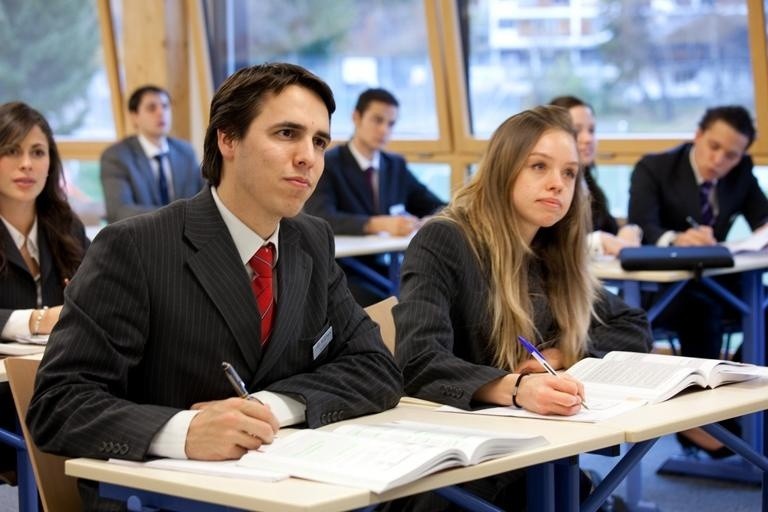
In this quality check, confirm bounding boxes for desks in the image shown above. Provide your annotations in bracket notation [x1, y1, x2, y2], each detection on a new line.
[400, 353, 766, 512]
[590, 247, 767, 482]
[64, 407, 628, 511]
[332, 231, 414, 298]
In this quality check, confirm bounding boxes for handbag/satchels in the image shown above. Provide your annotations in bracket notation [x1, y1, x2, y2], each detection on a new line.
[619, 245, 735, 270]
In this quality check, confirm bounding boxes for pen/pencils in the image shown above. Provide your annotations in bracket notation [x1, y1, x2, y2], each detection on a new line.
[222, 362, 253, 400]
[65, 279, 69, 283]
[518, 336, 590, 409]
[688, 216, 699, 228]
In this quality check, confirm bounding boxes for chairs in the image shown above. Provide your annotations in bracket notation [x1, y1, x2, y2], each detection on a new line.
[363, 295, 398, 356]
[2, 357, 83, 512]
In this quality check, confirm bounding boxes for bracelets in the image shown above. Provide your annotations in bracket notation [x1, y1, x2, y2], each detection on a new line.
[513, 373, 530, 409]
[34, 305, 48, 336]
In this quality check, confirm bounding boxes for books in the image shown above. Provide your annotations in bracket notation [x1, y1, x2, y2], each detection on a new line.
[560, 349, 762, 404]
[254, 410, 550, 498]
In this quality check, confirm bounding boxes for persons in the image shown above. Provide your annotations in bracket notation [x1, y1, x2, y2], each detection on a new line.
[548, 94, 747, 462]
[391, 106, 652, 419]
[300, 84, 454, 304]
[101, 79, 208, 231]
[24, 62, 407, 461]
[626, 103, 768, 366]
[0, 97, 98, 510]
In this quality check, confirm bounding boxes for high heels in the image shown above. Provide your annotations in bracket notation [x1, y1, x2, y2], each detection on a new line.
[676, 433, 734, 461]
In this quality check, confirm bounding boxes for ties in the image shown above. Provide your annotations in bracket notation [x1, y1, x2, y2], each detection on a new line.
[701, 181, 715, 225]
[154, 154, 170, 207]
[368, 168, 379, 213]
[248, 246, 274, 349]
